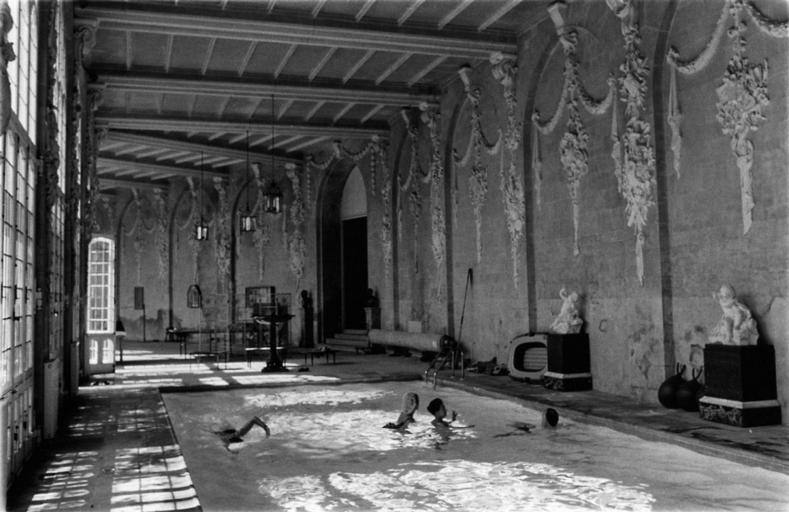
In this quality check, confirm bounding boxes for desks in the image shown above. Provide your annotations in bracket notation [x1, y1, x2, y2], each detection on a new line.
[171, 324, 271, 361]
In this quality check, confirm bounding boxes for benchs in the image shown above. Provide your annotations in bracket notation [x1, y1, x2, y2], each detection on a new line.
[298, 347, 341, 364]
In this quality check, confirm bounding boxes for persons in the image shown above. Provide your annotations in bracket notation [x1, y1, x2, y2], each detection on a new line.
[548, 286, 582, 336]
[425, 397, 476, 441]
[382, 389, 420, 435]
[539, 406, 568, 431]
[213, 415, 271, 454]
[716, 284, 754, 345]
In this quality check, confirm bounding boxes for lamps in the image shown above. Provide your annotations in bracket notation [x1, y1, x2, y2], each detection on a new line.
[194, 92, 283, 242]
